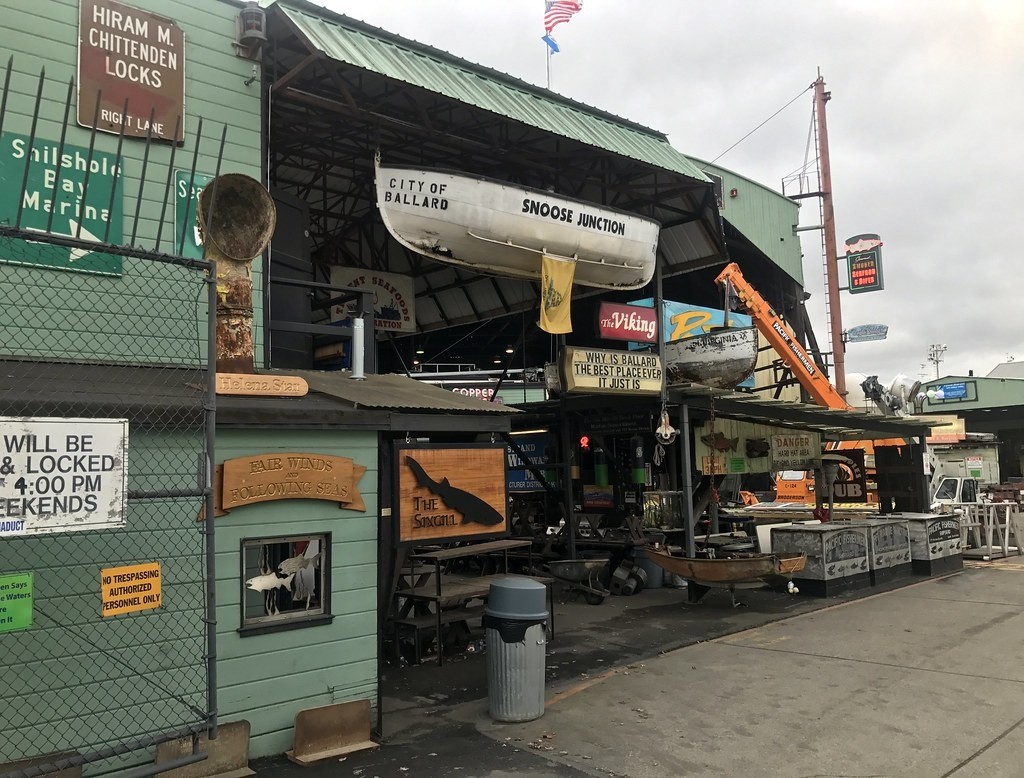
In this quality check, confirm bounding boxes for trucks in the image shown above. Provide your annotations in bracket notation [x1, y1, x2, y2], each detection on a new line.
[930, 476, 1018, 523]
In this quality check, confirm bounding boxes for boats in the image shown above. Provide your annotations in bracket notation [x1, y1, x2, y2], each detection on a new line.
[641, 545, 807, 581]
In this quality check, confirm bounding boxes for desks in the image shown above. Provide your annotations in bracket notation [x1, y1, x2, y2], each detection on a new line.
[868, 512, 963, 576]
[770, 527, 869, 598]
[831, 520, 913, 587]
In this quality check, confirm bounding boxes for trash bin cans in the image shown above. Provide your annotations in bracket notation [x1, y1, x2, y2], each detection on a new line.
[484, 577, 549, 723]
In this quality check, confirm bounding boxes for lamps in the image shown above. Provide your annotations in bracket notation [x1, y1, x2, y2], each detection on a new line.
[234, 3, 269, 59]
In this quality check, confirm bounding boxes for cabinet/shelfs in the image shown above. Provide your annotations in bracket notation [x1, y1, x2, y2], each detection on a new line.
[394, 540, 555, 666]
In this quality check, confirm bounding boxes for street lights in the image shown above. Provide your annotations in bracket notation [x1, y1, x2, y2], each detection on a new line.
[928, 344, 947, 379]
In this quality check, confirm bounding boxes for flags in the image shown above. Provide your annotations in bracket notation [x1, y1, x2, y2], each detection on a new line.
[330, 265, 416, 331]
[544, 0, 583, 32]
[536, 255, 576, 335]
